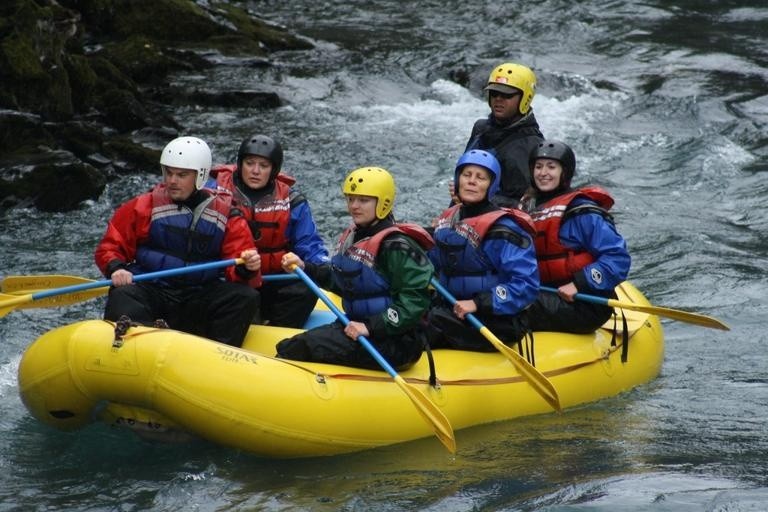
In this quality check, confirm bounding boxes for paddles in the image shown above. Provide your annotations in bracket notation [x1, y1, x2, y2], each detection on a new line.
[0, 255, 246, 320]
[292, 264, 457, 455]
[542, 288, 731, 332]
[432, 277, 563, 417]
[2, 273, 296, 308]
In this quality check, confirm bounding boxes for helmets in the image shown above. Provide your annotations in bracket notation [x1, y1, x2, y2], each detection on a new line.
[237, 132, 284, 188]
[342, 165, 397, 220]
[486, 62, 538, 115]
[454, 148, 500, 202]
[528, 140, 575, 183]
[159, 136, 213, 191]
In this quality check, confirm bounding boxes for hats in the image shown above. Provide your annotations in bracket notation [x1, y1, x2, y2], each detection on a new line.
[486, 82, 519, 95]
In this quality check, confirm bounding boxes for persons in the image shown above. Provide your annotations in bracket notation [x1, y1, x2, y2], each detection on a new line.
[203, 133, 333, 328]
[525, 141, 632, 364]
[274, 166, 437, 387]
[95, 135, 262, 350]
[448, 63, 547, 211]
[425, 150, 539, 371]
